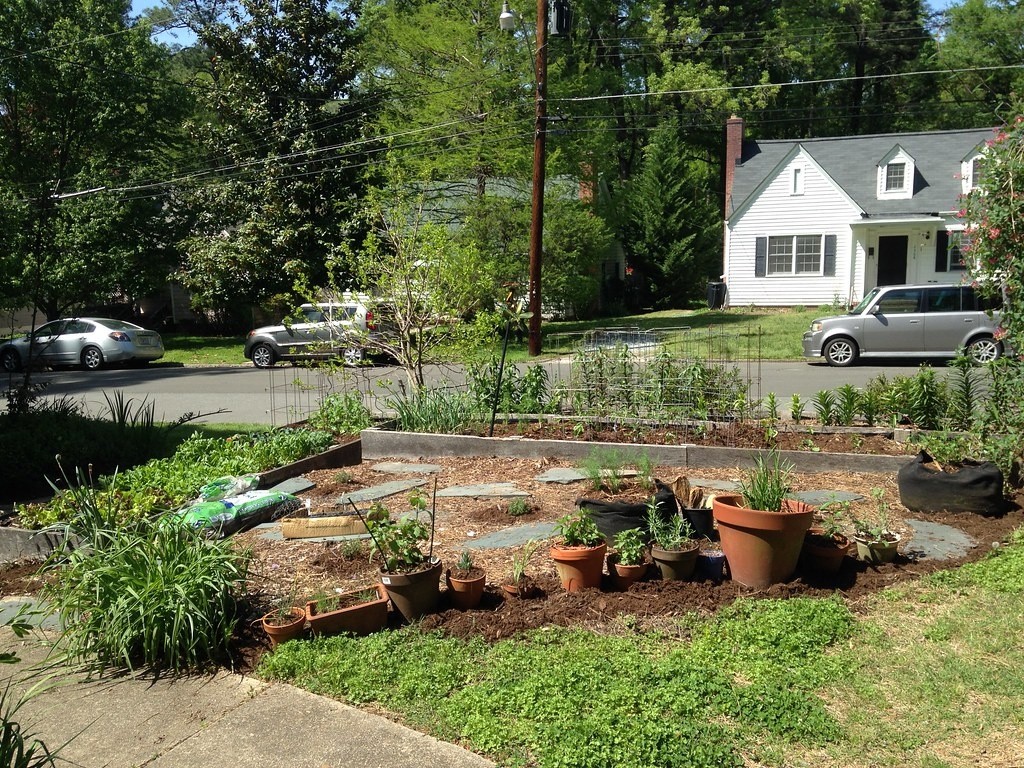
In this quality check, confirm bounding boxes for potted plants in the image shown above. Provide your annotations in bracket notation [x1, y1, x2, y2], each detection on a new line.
[446, 550, 487, 599]
[347, 477, 443, 625]
[549, 508, 608, 590]
[799, 494, 849, 585]
[675, 475, 714, 533]
[696, 532, 730, 581]
[502, 535, 550, 600]
[259, 571, 305, 645]
[608, 527, 653, 581]
[853, 484, 902, 570]
[306, 576, 390, 637]
[569, 445, 678, 549]
[647, 494, 700, 581]
[714, 443, 815, 587]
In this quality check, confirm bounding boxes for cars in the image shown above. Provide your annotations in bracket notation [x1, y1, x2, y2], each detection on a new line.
[0, 316, 165, 370]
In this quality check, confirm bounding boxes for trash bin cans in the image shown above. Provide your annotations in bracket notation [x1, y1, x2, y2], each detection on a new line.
[708, 281, 726, 310]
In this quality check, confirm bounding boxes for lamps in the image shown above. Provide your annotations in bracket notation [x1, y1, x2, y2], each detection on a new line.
[918, 230, 930, 240]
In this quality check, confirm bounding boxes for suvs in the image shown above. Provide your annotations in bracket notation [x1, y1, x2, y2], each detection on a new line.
[800, 278, 1014, 368]
[243, 303, 401, 370]
